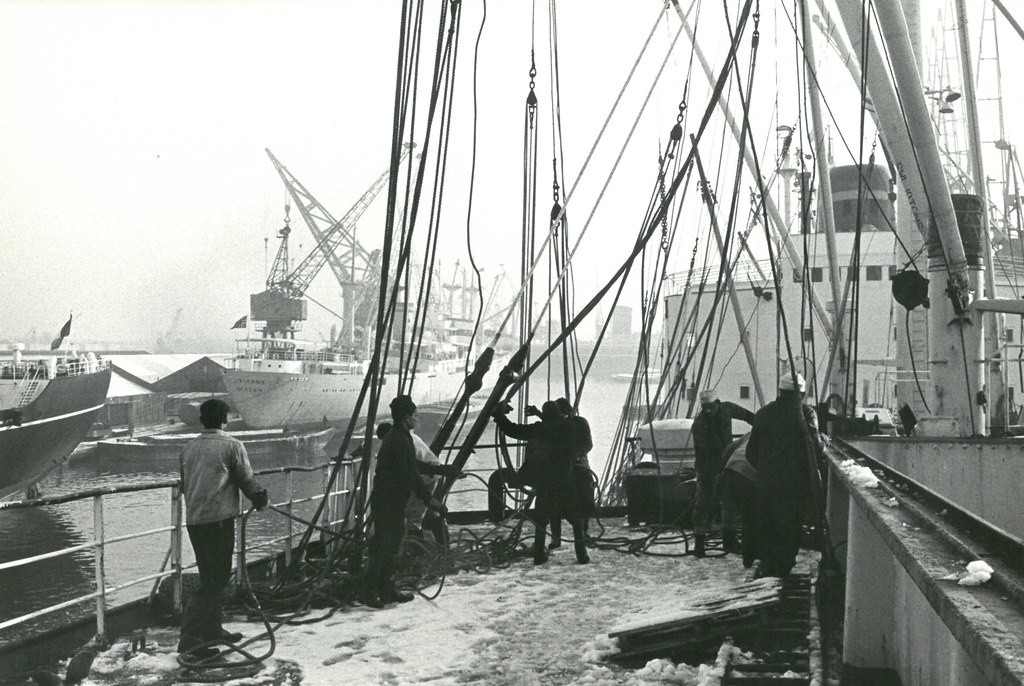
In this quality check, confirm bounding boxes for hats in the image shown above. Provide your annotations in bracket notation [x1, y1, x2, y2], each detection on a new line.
[779, 371, 807, 394]
[701, 391, 719, 405]
[200, 400, 231, 418]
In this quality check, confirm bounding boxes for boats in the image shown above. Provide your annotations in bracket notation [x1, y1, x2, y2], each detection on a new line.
[0, 346, 114, 509]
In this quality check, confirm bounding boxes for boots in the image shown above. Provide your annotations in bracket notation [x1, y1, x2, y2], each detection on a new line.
[723, 529, 741, 551]
[693, 534, 705, 557]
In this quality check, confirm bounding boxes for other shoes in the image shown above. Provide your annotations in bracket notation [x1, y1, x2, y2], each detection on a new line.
[204, 627, 243, 644]
[380, 588, 414, 603]
[549, 542, 560, 550]
[580, 556, 590, 564]
[535, 555, 549, 564]
[177, 642, 220, 658]
[357, 593, 385, 609]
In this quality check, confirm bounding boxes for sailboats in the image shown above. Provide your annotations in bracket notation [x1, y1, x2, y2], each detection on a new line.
[220, 0, 1022, 519]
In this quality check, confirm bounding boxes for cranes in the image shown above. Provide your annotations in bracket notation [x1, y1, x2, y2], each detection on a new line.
[248, 139, 420, 357]
[264, 146, 395, 332]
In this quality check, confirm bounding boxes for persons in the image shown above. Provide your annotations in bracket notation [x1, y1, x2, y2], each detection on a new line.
[177, 399, 270, 655]
[722, 372, 832, 566]
[485, 398, 596, 566]
[362, 395, 467, 603]
[691, 390, 757, 558]
